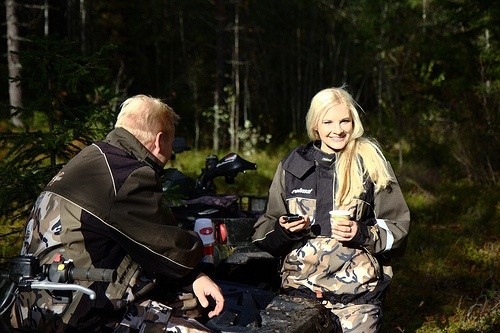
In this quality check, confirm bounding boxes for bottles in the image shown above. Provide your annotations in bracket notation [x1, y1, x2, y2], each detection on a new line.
[204, 244, 214, 264]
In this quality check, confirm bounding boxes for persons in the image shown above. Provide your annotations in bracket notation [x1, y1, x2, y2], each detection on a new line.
[247, 82, 410, 333]
[11, 94, 224, 333]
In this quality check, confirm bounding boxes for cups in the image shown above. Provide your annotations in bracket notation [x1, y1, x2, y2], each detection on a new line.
[193, 217, 216, 246]
[329, 210, 352, 239]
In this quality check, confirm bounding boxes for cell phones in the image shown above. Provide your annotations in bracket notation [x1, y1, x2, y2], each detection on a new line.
[283, 216, 302, 222]
